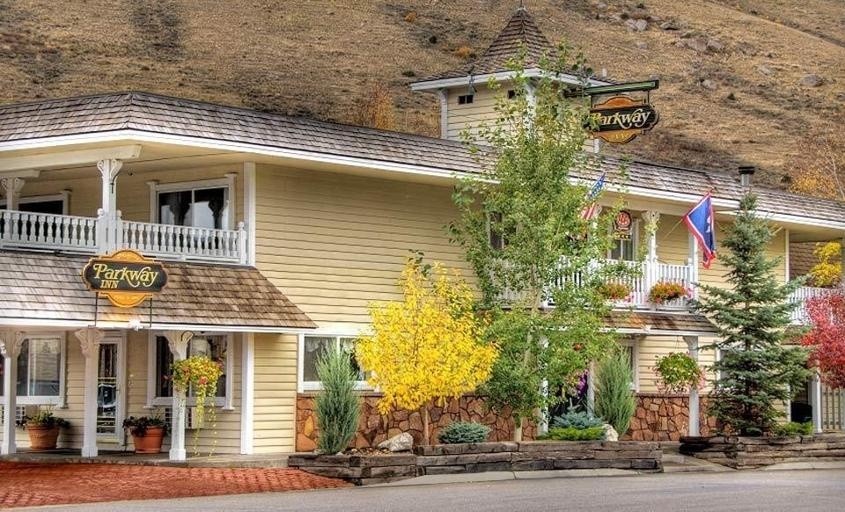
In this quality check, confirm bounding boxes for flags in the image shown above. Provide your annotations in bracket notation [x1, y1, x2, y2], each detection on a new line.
[566, 174, 605, 240]
[682, 192, 717, 268]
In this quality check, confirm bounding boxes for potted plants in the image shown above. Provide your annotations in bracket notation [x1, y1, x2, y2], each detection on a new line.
[17, 407, 71, 449]
[120, 408, 168, 454]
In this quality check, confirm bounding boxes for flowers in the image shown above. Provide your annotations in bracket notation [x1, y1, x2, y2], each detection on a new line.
[596, 282, 636, 302]
[647, 282, 686, 308]
[166, 355, 225, 458]
